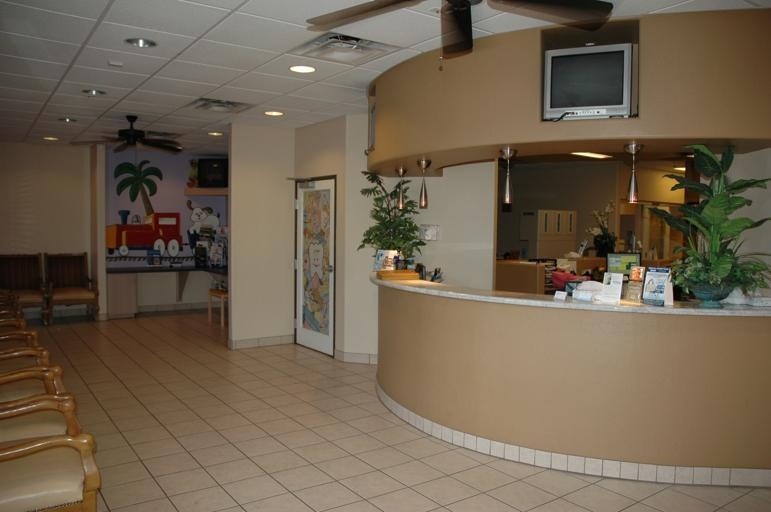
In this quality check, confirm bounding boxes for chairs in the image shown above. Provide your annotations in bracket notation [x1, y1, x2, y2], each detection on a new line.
[1, 246, 99, 511]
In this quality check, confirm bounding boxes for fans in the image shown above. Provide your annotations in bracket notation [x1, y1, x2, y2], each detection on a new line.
[68, 114, 187, 156]
[304, 0, 615, 61]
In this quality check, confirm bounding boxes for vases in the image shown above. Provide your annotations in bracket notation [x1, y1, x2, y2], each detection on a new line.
[593, 241, 616, 258]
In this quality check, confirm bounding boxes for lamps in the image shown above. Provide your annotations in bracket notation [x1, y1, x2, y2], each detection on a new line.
[395, 159, 429, 213]
[502, 148, 518, 206]
[624, 143, 645, 206]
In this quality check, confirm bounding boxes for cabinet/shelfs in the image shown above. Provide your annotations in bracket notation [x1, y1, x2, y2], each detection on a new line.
[516, 208, 578, 259]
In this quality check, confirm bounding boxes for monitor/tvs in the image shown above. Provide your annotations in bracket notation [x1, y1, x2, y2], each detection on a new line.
[543, 43, 639, 120]
[606, 252, 640, 282]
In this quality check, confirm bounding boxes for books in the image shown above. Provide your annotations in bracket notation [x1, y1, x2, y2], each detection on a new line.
[376, 270, 420, 280]
[577, 240, 588, 257]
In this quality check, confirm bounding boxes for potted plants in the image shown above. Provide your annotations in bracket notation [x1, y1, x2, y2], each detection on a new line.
[646, 141, 771, 303]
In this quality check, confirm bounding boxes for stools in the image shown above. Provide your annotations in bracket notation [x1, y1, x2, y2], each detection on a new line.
[207, 287, 229, 329]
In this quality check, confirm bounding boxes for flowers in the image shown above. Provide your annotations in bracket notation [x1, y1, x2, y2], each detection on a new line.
[584, 197, 618, 247]
